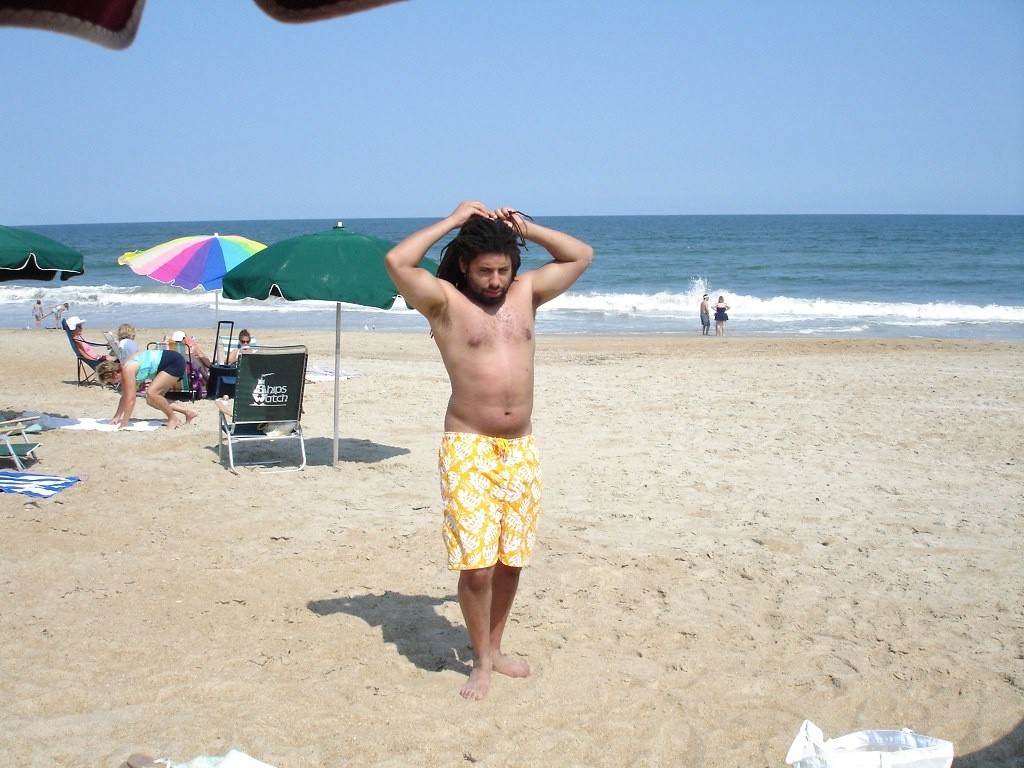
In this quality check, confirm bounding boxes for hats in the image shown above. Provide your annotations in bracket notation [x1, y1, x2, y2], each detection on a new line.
[173, 331, 186, 342]
[66, 316, 86, 330]
[703, 295, 710, 298]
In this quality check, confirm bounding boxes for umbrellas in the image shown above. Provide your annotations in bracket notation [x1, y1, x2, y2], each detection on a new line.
[0, 224, 84, 284]
[222, 222, 441, 468]
[118, 232, 268, 330]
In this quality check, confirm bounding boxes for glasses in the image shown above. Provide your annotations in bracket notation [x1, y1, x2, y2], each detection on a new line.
[240, 340, 250, 343]
[76, 325, 81, 328]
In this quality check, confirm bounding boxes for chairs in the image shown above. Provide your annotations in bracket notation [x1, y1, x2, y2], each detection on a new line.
[61, 318, 121, 387]
[102, 332, 149, 398]
[216, 335, 257, 366]
[0, 414, 43, 472]
[217, 345, 306, 475]
[146, 342, 202, 404]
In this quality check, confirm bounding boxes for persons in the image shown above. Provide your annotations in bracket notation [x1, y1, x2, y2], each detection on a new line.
[66, 316, 116, 362]
[38, 303, 68, 329]
[225, 329, 258, 366]
[95, 350, 198, 432]
[385, 202, 593, 702]
[164, 331, 224, 386]
[32, 299, 44, 329]
[700, 293, 712, 336]
[711, 296, 730, 336]
[109, 323, 142, 363]
